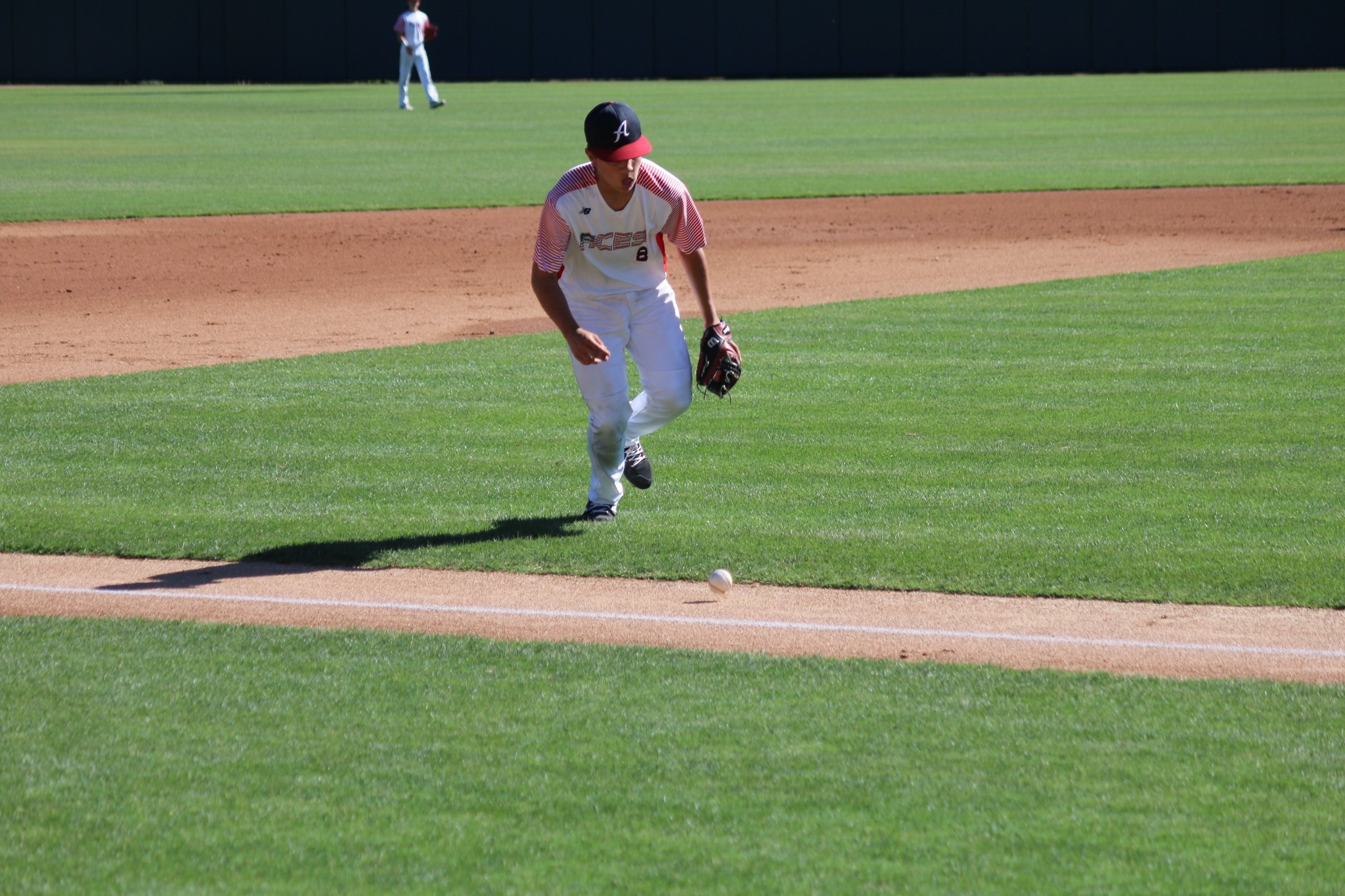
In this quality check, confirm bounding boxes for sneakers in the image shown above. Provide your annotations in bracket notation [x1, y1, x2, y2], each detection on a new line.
[623, 441, 652, 489]
[583, 499, 615, 521]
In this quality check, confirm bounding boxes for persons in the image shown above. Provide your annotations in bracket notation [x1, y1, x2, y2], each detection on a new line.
[393, 0, 446, 111]
[531, 101, 742, 520]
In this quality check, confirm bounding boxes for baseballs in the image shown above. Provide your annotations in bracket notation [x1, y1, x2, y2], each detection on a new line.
[708, 569, 733, 594]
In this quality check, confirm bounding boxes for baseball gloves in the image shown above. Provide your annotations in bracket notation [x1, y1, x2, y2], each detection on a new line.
[425, 24, 438, 41]
[695, 315, 742, 405]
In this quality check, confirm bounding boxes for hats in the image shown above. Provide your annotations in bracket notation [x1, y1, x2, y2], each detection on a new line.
[584, 101, 652, 162]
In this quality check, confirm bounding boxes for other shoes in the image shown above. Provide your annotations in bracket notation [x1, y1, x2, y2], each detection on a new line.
[400, 105, 413, 110]
[431, 99, 446, 109]
